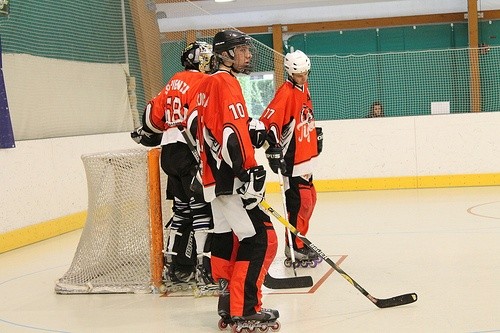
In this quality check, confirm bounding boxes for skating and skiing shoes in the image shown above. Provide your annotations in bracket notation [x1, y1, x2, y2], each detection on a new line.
[230, 307, 282, 333]
[217, 278, 235, 330]
[194, 263, 224, 296]
[284, 243, 322, 268]
[160, 256, 198, 294]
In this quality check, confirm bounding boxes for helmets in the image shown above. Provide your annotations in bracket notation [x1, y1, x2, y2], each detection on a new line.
[213, 29, 258, 76]
[181, 41, 215, 73]
[283, 50, 311, 77]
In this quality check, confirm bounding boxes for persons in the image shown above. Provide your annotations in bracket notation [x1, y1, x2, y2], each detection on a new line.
[363, 102, 384, 117]
[197, 31, 280, 333]
[131, 40, 266, 297]
[259, 50, 322, 268]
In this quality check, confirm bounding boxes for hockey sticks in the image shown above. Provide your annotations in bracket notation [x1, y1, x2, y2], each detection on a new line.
[278, 162, 299, 278]
[261, 199, 418, 309]
[176, 122, 314, 290]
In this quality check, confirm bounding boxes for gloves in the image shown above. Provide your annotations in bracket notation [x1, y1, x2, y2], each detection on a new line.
[247, 117, 266, 149]
[236, 166, 267, 210]
[264, 146, 287, 175]
[130, 126, 163, 146]
[190, 160, 203, 193]
[315, 127, 323, 154]
